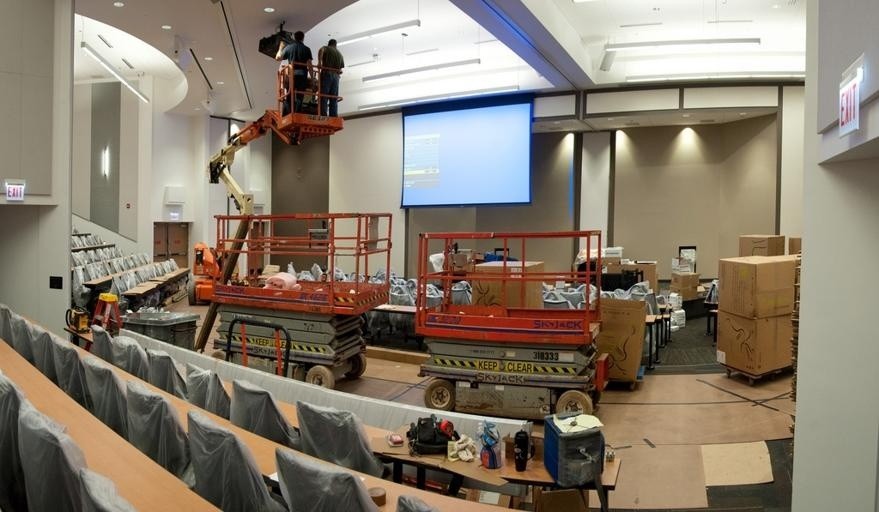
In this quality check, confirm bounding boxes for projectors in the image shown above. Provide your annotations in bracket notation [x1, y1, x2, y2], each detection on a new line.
[258, 31, 294, 61]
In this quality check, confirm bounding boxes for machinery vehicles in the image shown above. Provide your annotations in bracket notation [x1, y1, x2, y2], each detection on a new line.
[409, 229, 606, 418]
[206, 59, 393, 391]
[184, 242, 239, 305]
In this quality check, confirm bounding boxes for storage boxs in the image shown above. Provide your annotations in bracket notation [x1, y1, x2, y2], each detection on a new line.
[593, 234, 802, 382]
[464, 485, 592, 511]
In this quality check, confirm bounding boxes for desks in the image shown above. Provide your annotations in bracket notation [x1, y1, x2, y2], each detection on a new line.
[2, 342, 231, 509]
[176, 364, 622, 509]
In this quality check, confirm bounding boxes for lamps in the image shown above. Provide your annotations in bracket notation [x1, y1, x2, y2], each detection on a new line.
[80, 24, 150, 105]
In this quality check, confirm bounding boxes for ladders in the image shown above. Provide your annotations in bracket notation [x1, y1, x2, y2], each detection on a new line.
[82, 293, 121, 354]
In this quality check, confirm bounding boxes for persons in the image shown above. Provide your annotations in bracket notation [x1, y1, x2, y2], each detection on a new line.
[275, 31, 345, 117]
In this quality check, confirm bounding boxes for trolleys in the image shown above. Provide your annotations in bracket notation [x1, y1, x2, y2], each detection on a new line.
[606, 364, 647, 391]
[719, 357, 794, 386]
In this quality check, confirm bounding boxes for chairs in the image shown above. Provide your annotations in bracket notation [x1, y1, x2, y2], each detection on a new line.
[393, 495, 435, 509]
[69, 235, 188, 312]
[274, 445, 383, 511]
[186, 362, 231, 420]
[296, 399, 390, 480]
[232, 379, 302, 453]
[186, 410, 287, 510]
[290, 262, 686, 377]
[2, 305, 187, 509]
[704, 281, 720, 346]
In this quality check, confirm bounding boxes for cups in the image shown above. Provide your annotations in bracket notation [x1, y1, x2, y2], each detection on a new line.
[369, 487, 388, 507]
[512, 429, 529, 472]
